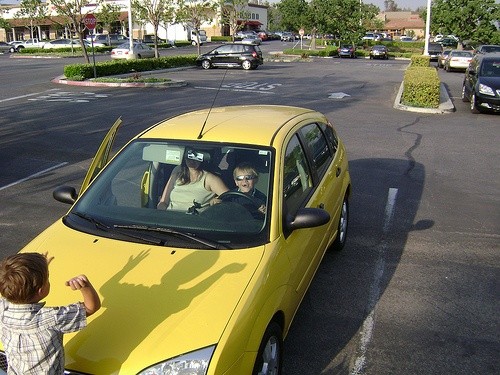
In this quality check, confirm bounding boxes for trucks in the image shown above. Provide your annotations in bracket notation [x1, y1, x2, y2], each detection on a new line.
[145, 22, 207, 46]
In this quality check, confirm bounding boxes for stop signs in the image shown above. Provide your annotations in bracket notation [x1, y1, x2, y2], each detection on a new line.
[85, 14, 98, 30]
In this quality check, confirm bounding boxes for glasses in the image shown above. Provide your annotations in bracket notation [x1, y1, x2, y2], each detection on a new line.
[235, 174, 255, 181]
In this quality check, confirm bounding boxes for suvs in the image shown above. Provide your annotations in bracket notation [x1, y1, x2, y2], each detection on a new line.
[196, 42, 264, 71]
[143, 35, 168, 43]
[461, 51, 500, 115]
[87, 34, 130, 46]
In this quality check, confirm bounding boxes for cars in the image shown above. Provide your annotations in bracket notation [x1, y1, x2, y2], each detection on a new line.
[42, 38, 89, 50]
[361, 31, 389, 42]
[369, 46, 389, 60]
[337, 44, 357, 58]
[75, 39, 105, 48]
[0, 104, 351, 375]
[7, 40, 23, 45]
[474, 45, 500, 57]
[399, 36, 414, 42]
[423, 34, 475, 72]
[236, 30, 334, 44]
[0, 41, 16, 54]
[244, 35, 262, 45]
[110, 41, 161, 61]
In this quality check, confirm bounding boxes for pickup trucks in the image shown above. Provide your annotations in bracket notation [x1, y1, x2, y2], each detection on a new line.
[12, 38, 46, 53]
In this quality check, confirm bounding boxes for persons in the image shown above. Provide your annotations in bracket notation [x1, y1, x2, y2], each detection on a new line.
[156, 146, 229, 214]
[0, 252, 101, 375]
[209, 162, 270, 222]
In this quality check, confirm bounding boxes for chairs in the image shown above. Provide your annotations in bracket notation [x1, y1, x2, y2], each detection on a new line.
[256, 169, 268, 195]
[194, 149, 222, 177]
